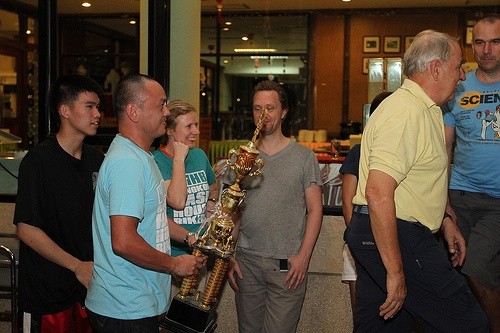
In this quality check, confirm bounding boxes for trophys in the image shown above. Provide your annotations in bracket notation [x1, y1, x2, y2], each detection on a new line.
[158, 103, 269, 332]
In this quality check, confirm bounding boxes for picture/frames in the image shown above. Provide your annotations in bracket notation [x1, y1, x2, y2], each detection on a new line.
[362, 57, 383, 75]
[362, 35, 381, 54]
[384, 36, 402, 54]
[384, 57, 401, 75]
[404, 36, 416, 53]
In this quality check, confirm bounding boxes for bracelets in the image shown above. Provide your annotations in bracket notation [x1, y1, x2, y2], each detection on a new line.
[206, 197, 217, 201]
[443, 213, 452, 221]
[183, 232, 194, 243]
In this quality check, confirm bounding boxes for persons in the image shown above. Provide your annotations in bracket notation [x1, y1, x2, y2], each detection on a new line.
[338, 13, 500, 332]
[13, 72, 324, 332]
